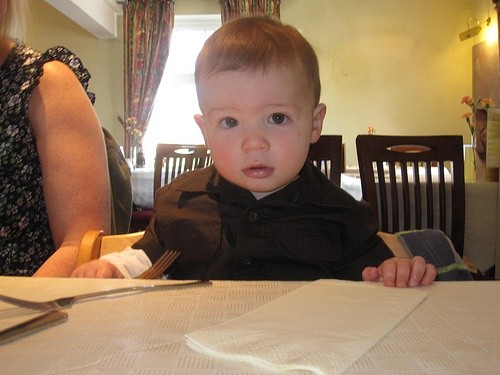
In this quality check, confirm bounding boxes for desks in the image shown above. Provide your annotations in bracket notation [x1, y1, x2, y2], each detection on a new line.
[119, 166, 451, 238]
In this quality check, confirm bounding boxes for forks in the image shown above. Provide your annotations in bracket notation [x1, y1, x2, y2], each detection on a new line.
[0, 280, 213, 319]
[134, 249, 181, 279]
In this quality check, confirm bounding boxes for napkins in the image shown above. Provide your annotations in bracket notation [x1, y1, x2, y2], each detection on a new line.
[184, 278, 428, 375]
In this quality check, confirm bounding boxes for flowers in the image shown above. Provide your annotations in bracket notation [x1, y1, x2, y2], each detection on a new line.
[125, 116, 143, 144]
[460, 95, 496, 135]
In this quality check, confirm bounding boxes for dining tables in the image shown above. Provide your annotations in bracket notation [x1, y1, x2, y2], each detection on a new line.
[0, 276, 500, 375]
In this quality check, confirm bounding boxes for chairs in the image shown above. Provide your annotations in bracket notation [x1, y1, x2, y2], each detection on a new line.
[356, 135, 465, 260]
[154, 144, 207, 212]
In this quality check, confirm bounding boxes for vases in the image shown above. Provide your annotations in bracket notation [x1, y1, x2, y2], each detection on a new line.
[136, 144, 145, 168]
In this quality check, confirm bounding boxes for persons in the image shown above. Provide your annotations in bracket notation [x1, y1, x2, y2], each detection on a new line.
[0, 0, 113, 281]
[69, 12, 437, 289]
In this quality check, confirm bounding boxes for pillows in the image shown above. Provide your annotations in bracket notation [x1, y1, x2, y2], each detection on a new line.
[394, 229, 473, 281]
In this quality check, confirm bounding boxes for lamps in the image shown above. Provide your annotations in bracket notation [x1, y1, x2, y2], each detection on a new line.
[459, 17, 482, 42]
